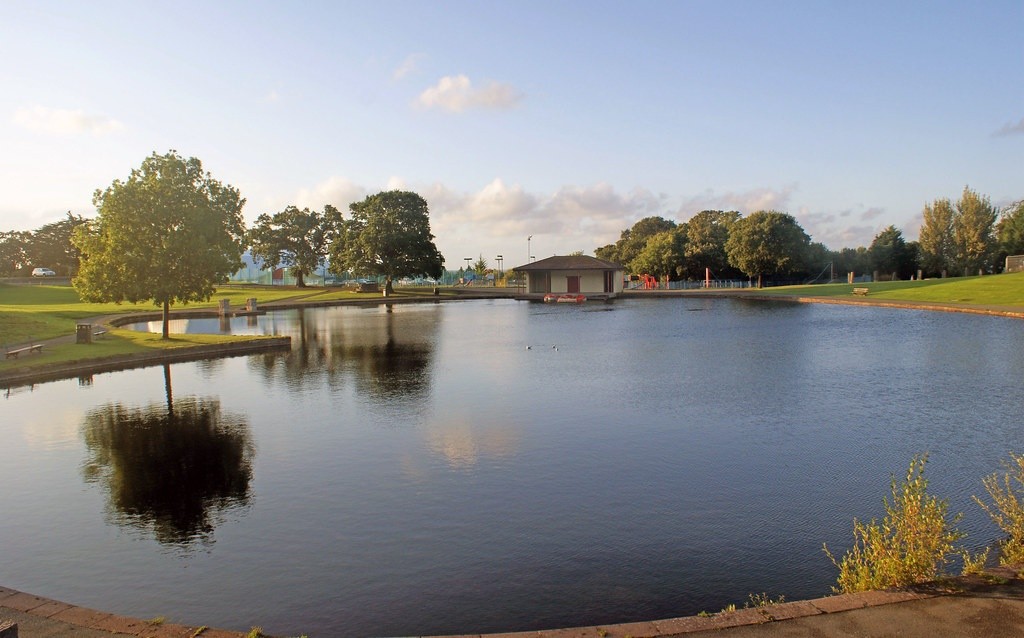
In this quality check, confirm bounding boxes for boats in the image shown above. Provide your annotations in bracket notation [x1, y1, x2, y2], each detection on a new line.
[545, 294, 586, 303]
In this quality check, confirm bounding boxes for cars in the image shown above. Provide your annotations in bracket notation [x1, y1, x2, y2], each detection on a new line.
[31, 267, 56, 277]
